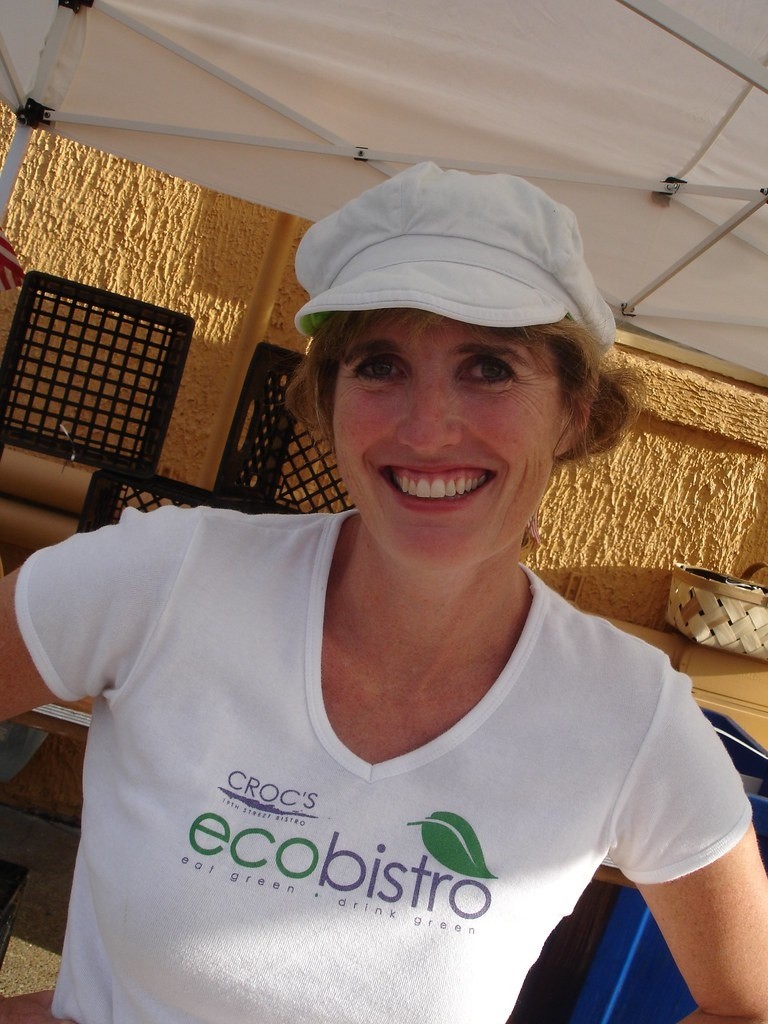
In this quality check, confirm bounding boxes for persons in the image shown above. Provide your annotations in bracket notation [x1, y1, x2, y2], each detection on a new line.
[0, 161, 768, 1024]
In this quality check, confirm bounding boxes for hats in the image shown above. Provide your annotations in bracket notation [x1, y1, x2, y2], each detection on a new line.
[295, 161, 615, 362]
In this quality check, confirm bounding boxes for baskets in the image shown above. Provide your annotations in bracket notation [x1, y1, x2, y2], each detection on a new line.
[667, 565, 768, 660]
